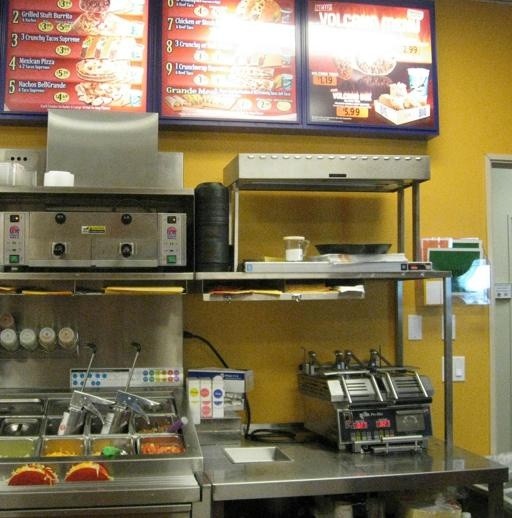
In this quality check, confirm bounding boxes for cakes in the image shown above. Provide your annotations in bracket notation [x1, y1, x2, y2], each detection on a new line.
[66, 461, 112, 482]
[8, 465, 58, 486]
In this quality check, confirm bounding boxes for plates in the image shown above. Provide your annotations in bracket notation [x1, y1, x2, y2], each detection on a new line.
[315, 243, 393, 254]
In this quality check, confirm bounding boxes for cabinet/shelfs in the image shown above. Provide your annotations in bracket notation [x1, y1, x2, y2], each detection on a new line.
[0, 187, 195, 285]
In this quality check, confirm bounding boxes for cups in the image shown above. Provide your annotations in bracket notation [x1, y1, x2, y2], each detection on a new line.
[282, 235, 310, 262]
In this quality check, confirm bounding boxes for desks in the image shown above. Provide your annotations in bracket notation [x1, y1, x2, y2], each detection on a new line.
[195, 152, 509, 518]
